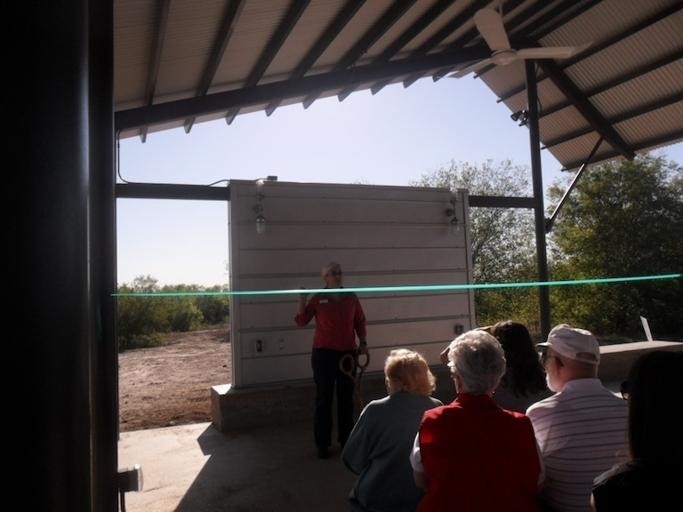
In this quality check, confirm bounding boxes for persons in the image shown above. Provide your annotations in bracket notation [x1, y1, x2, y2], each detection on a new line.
[408, 327, 547, 509]
[294, 258, 368, 458]
[490, 320, 556, 413]
[590, 347, 683, 511]
[339, 346, 443, 509]
[520, 322, 634, 510]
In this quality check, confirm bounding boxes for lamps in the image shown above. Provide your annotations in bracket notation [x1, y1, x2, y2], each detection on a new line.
[511, 110, 529, 127]
[448, 198, 460, 240]
[253, 193, 266, 234]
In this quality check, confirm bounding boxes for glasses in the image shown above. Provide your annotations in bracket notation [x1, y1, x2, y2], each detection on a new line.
[541, 348, 565, 367]
[326, 270, 342, 277]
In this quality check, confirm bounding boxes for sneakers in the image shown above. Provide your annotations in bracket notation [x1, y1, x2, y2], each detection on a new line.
[317, 442, 347, 459]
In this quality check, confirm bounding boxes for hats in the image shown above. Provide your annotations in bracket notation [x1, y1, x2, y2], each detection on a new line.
[533, 323, 601, 365]
[320, 259, 342, 282]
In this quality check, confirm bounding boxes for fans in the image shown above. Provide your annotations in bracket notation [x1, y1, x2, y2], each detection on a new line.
[448, 0, 575, 78]
